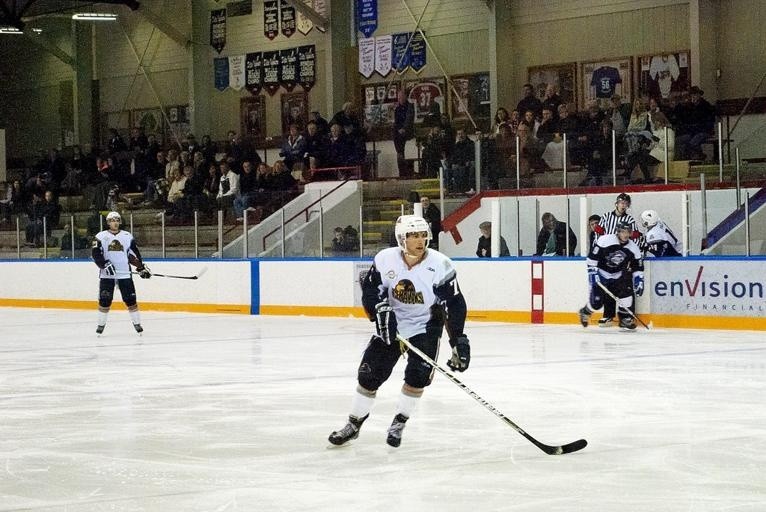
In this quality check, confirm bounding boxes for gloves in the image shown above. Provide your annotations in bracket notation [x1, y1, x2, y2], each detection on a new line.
[101, 260, 115, 275]
[588, 267, 601, 287]
[375, 302, 397, 346]
[447, 334, 470, 372]
[137, 264, 152, 278]
[633, 271, 644, 296]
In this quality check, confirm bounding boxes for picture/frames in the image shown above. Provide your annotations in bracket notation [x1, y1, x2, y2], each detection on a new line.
[239, 95, 266, 138]
[637, 50, 691, 101]
[580, 55, 634, 110]
[102, 103, 190, 146]
[450, 72, 491, 123]
[280, 92, 309, 133]
[527, 62, 577, 110]
[360, 80, 401, 131]
[403, 75, 447, 127]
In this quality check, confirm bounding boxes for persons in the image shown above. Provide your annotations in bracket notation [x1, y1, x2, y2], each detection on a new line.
[579, 221, 643, 329]
[91, 212, 152, 334]
[594, 192, 642, 323]
[588, 214, 601, 256]
[390, 82, 716, 199]
[476, 221, 510, 256]
[1, 102, 367, 256]
[327, 214, 471, 448]
[640, 210, 683, 256]
[533, 212, 577, 257]
[390, 191, 441, 251]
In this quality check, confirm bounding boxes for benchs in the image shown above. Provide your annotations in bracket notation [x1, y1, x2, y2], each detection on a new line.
[1, 170, 304, 259]
[403, 138, 747, 197]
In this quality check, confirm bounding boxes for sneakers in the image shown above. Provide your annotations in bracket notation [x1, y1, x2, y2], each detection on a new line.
[97, 326, 104, 334]
[134, 324, 143, 332]
[157, 211, 174, 223]
[580, 305, 636, 329]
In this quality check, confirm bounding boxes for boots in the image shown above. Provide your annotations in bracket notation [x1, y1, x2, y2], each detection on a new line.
[387, 413, 409, 447]
[329, 413, 369, 445]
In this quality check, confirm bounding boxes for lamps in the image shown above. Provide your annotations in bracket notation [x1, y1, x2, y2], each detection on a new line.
[0, 27, 44, 35]
[71, 12, 119, 21]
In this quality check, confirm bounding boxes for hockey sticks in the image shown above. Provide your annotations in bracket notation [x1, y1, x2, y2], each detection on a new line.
[597, 280, 670, 334]
[114, 264, 208, 279]
[396, 331, 587, 455]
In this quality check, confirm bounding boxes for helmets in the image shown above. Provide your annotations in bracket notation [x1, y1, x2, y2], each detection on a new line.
[616, 221, 631, 232]
[617, 193, 630, 203]
[106, 211, 122, 222]
[395, 215, 433, 250]
[641, 210, 657, 226]
